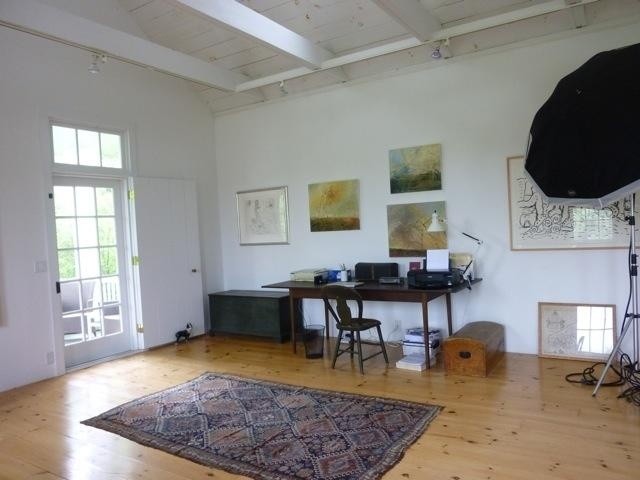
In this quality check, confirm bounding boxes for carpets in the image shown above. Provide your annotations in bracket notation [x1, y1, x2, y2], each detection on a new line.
[80, 371, 447, 480]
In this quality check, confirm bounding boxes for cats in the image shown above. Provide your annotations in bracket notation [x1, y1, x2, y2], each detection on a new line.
[175, 322, 193, 345]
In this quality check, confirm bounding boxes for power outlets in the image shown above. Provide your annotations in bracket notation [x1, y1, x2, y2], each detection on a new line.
[395, 320, 402, 330]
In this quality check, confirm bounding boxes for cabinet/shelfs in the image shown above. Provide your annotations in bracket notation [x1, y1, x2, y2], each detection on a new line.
[208, 289, 302, 344]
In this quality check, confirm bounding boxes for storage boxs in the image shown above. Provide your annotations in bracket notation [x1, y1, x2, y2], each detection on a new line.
[444, 321, 505, 378]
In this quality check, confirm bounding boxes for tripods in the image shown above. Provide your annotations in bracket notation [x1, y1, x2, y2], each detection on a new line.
[591, 193, 640, 398]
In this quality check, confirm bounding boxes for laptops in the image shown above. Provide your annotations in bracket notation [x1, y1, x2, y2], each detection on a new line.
[325, 280, 365, 288]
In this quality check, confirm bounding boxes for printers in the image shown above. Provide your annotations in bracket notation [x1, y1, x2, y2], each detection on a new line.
[402, 328, 441, 360]
[407, 259, 462, 289]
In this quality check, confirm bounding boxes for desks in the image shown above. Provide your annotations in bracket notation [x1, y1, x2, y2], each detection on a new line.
[261, 274, 483, 370]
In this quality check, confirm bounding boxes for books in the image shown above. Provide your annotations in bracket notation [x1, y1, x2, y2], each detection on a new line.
[396, 352, 436, 371]
[290, 268, 329, 282]
[327, 281, 365, 287]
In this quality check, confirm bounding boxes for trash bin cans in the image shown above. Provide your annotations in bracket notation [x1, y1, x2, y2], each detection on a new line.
[302, 324, 326, 358]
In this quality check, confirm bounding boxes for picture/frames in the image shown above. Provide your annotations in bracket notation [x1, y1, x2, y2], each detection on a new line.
[236, 185, 290, 246]
[537, 301, 617, 365]
[506, 155, 639, 252]
[308, 180, 361, 232]
[389, 143, 442, 195]
[386, 200, 447, 257]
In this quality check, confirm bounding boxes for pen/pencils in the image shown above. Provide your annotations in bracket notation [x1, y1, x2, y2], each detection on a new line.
[340, 264, 347, 270]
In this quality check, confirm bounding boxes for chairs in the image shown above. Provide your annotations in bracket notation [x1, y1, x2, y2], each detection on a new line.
[321, 285, 389, 375]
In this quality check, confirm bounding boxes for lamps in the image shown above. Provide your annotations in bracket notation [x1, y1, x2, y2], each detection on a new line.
[426, 210, 485, 289]
[431, 44, 441, 58]
[279, 87, 288, 98]
[88, 56, 100, 75]
